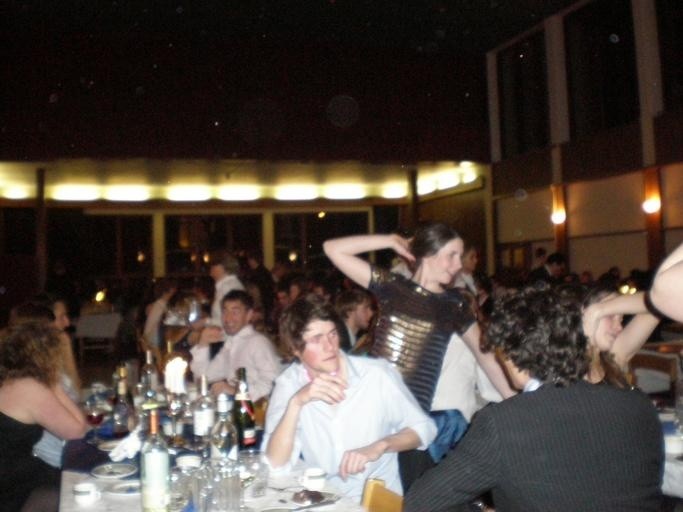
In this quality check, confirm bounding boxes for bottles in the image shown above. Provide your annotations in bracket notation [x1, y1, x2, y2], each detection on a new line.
[73, 343, 349, 511]
[167, 246, 256, 271]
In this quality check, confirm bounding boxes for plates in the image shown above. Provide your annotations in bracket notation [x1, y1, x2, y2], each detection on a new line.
[650, 388, 683, 462]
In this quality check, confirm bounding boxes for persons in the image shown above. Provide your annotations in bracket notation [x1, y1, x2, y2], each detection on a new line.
[1, 216, 683, 512]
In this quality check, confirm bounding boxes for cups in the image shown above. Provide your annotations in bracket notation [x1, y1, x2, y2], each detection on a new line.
[163, 297, 214, 322]
